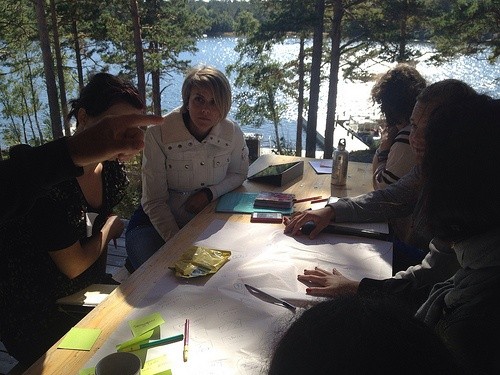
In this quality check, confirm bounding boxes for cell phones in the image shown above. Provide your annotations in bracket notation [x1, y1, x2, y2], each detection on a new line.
[250, 212, 283, 223]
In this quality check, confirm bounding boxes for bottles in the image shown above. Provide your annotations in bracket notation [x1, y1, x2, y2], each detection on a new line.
[330, 138, 348, 186]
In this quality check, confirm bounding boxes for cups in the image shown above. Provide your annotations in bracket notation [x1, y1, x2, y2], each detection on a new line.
[95, 351, 142, 375]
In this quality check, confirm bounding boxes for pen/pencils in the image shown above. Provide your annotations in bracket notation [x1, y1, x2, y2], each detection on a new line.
[117, 333, 183, 354]
[294, 199, 297, 201]
[244, 283, 297, 311]
[183, 319, 190, 362]
[113, 236, 118, 248]
[311, 198, 329, 204]
[294, 196, 322, 203]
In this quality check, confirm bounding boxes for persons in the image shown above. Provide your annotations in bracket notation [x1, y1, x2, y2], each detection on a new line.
[124, 64, 249, 270]
[262, 295, 459, 375]
[0, 72, 148, 364]
[0, 112, 168, 224]
[282, 63, 500, 375]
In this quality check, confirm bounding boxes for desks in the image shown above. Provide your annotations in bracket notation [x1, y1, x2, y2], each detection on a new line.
[22, 154, 393, 375]
